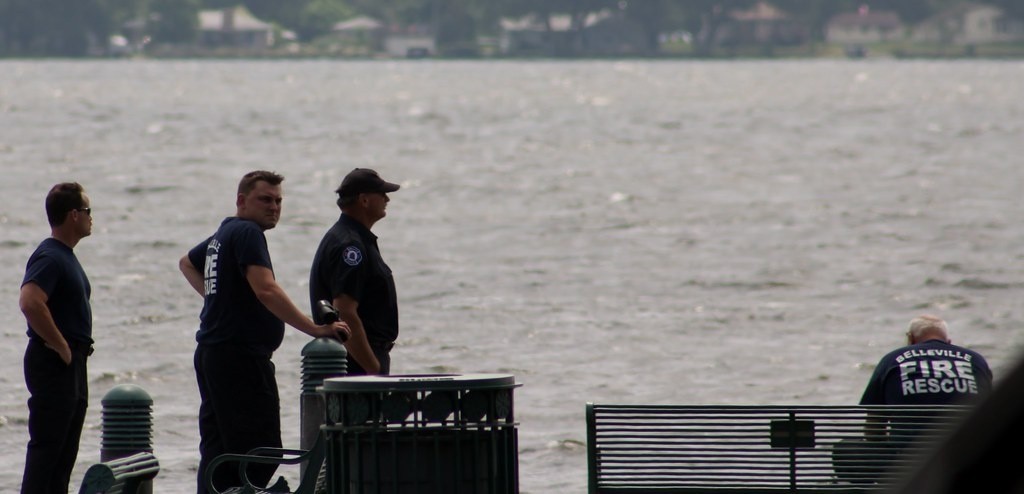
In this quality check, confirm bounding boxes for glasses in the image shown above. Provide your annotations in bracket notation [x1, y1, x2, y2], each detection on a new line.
[76, 207, 91, 215]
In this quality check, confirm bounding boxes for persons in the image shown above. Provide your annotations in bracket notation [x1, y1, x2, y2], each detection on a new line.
[179, 170, 351, 494]
[310, 169, 400, 375]
[20, 183, 95, 494]
[831, 314, 993, 488]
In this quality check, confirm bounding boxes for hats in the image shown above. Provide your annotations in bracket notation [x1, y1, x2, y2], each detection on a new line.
[339, 168, 400, 197]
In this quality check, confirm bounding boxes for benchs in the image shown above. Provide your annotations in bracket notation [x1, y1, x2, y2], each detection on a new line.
[205, 387, 330, 494]
[586, 402, 977, 494]
[78, 452, 161, 494]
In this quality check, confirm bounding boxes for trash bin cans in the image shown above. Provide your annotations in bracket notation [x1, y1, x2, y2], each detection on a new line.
[315, 372, 522, 493]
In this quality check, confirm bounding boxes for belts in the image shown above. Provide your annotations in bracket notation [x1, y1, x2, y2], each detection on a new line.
[367, 341, 393, 352]
